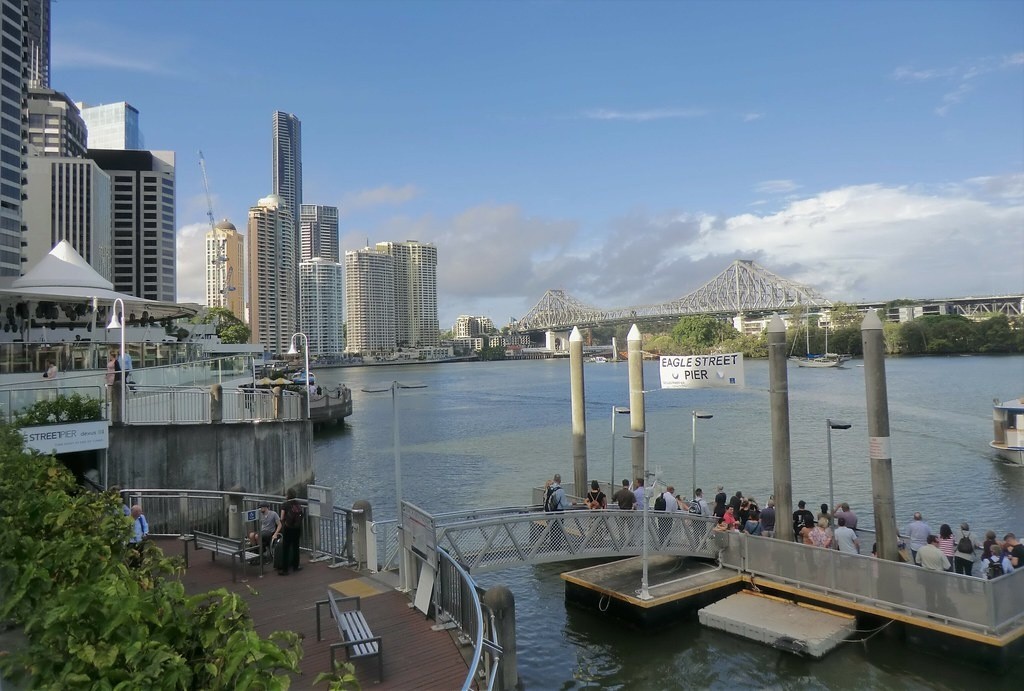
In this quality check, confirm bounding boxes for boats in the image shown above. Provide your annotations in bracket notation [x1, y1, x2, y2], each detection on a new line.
[291, 371, 316, 385]
[584, 356, 608, 363]
[841, 354, 853, 362]
[992, 398, 1024, 467]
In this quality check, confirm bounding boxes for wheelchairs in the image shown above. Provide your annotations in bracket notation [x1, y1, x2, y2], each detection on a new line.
[238, 533, 282, 560]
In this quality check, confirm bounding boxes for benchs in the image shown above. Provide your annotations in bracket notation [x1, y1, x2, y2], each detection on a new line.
[316, 589, 383, 682]
[185, 530, 263, 582]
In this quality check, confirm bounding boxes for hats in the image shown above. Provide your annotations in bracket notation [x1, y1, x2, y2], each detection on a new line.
[258, 500, 267, 507]
[798, 501, 806, 507]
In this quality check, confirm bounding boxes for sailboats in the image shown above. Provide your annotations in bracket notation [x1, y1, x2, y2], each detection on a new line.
[786, 302, 845, 367]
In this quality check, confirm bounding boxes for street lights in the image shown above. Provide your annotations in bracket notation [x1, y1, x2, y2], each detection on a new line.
[611, 406, 631, 504]
[362, 379, 428, 590]
[106, 298, 125, 423]
[826, 418, 852, 548]
[288, 333, 311, 419]
[691, 411, 713, 500]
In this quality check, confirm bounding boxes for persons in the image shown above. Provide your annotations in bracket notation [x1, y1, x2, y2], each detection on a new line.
[107, 351, 133, 401]
[250, 488, 303, 575]
[310, 385, 322, 396]
[47, 360, 57, 379]
[542, 474, 1024, 635]
[104, 485, 149, 573]
[337, 383, 345, 399]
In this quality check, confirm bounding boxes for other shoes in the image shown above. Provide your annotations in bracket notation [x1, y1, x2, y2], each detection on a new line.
[294, 566, 302, 571]
[278, 571, 288, 575]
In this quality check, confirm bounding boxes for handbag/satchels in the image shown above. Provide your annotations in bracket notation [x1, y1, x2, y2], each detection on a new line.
[127, 374, 135, 390]
[43, 372, 48, 377]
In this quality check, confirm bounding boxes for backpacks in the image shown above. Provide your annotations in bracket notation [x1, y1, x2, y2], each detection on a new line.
[285, 501, 303, 527]
[955, 529, 976, 557]
[689, 499, 701, 514]
[589, 492, 601, 512]
[543, 486, 562, 512]
[654, 492, 666, 510]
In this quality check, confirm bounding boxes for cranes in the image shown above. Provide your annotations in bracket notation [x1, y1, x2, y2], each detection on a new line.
[197, 150, 236, 310]
